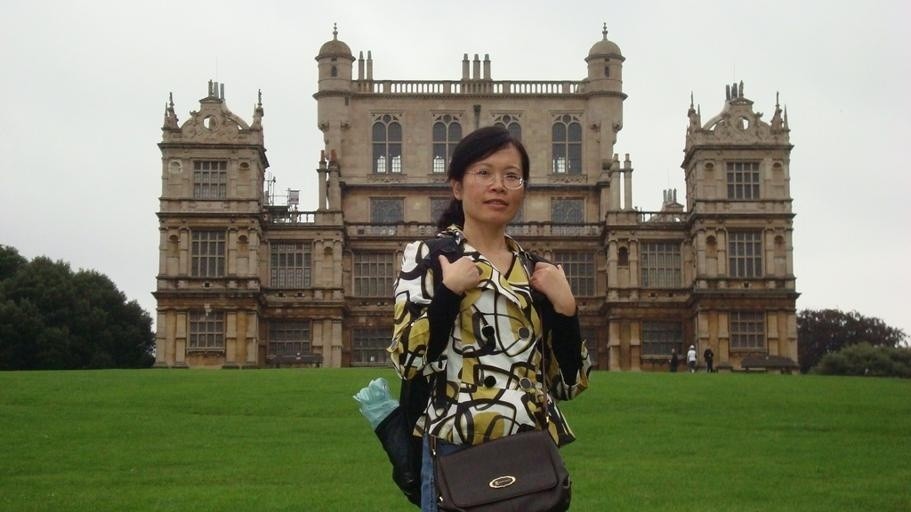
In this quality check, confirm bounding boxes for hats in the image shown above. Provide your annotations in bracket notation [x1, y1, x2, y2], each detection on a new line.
[690, 346, 695, 349]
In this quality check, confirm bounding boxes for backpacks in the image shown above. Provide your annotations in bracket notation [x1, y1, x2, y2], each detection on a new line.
[375, 237, 554, 506]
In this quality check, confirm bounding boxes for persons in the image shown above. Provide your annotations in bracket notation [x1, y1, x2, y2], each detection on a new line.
[668, 348, 679, 372]
[704, 348, 713, 372]
[686, 344, 698, 373]
[385, 124, 595, 512]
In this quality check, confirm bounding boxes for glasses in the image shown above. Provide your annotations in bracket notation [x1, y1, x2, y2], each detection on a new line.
[466, 168, 524, 190]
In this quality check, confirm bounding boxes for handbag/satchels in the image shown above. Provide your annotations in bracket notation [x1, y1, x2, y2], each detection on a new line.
[435, 429, 571, 512]
